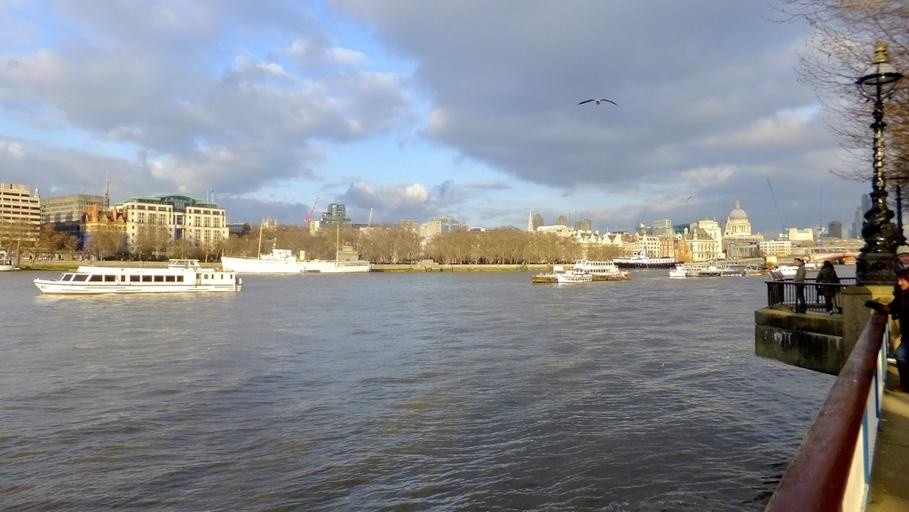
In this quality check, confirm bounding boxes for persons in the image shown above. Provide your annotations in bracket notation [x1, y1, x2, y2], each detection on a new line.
[767, 263, 784, 303]
[815, 260, 840, 314]
[886, 244, 909, 363]
[794, 258, 808, 314]
[864, 270, 909, 393]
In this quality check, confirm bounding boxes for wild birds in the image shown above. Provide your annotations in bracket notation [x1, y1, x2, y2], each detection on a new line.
[577, 98, 618, 106]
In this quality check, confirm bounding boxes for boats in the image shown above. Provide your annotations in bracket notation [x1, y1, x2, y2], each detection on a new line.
[531, 259, 630, 283]
[669, 262, 762, 279]
[33, 258, 243, 294]
[220, 257, 372, 273]
[767, 265, 817, 276]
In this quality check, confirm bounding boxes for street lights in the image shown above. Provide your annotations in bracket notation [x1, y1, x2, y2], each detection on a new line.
[856, 43, 898, 284]
